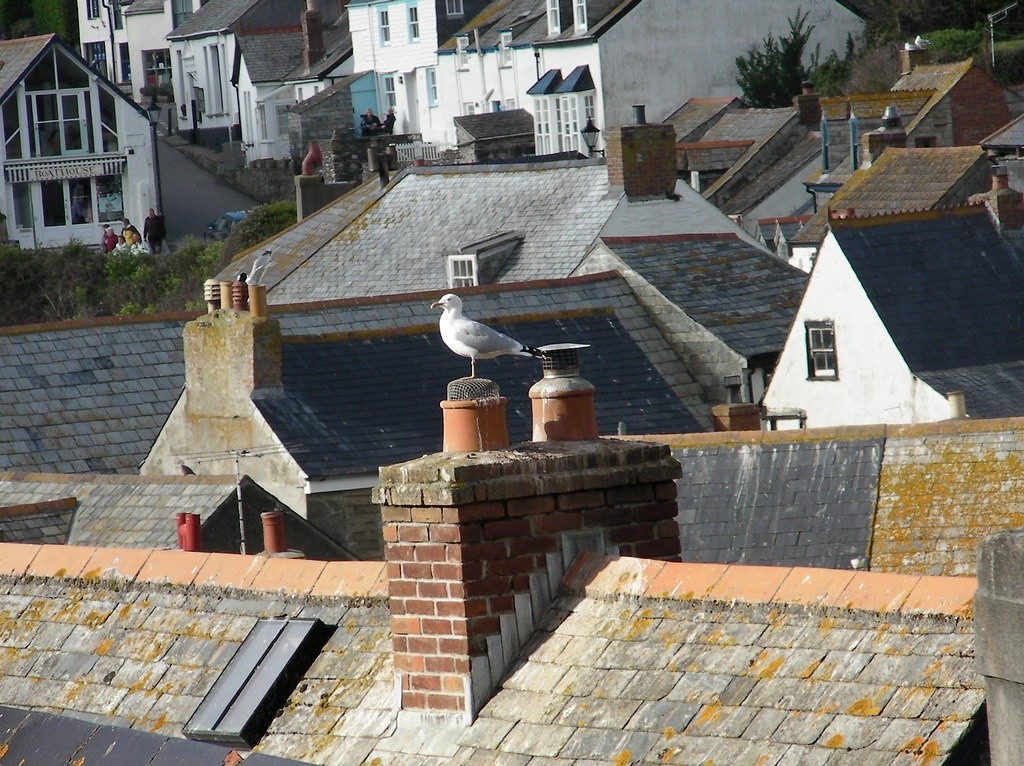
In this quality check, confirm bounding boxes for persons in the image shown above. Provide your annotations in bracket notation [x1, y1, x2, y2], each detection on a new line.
[101, 224, 111, 251]
[380, 106, 397, 135]
[104, 227, 120, 253]
[129, 232, 151, 257]
[112, 235, 130, 257]
[143, 207, 170, 255]
[120, 218, 138, 247]
[360, 108, 382, 137]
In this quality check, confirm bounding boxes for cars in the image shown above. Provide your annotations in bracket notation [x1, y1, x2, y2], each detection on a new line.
[205, 211, 255, 249]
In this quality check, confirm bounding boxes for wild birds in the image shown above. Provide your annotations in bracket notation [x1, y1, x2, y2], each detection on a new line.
[430, 293, 546, 380]
[904, 42, 920, 50]
[237, 250, 274, 286]
[915, 35, 931, 48]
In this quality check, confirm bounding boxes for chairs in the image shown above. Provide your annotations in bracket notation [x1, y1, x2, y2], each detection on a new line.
[360, 114, 368, 136]
[379, 116, 396, 135]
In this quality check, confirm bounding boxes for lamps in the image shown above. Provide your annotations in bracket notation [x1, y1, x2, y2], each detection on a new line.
[578, 118, 607, 161]
